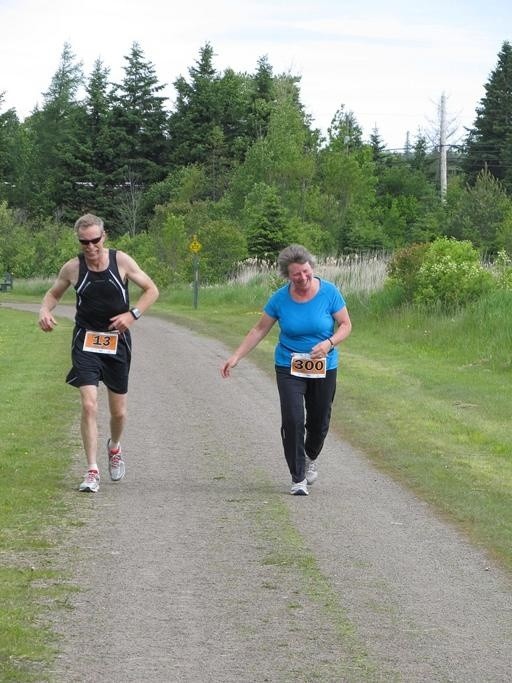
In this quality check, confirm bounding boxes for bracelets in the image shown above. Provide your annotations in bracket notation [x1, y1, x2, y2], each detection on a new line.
[129, 306, 143, 320]
[327, 336, 335, 349]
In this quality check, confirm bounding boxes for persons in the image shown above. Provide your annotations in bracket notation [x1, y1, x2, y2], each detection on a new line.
[219, 242, 354, 495]
[38, 210, 160, 495]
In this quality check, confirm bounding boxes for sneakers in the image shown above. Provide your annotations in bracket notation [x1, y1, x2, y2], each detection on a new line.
[290, 478, 309, 495]
[79, 469, 100, 492]
[107, 438, 125, 482]
[304, 454, 317, 484]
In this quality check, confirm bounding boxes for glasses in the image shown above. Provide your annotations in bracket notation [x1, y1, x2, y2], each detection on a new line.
[78, 233, 103, 245]
